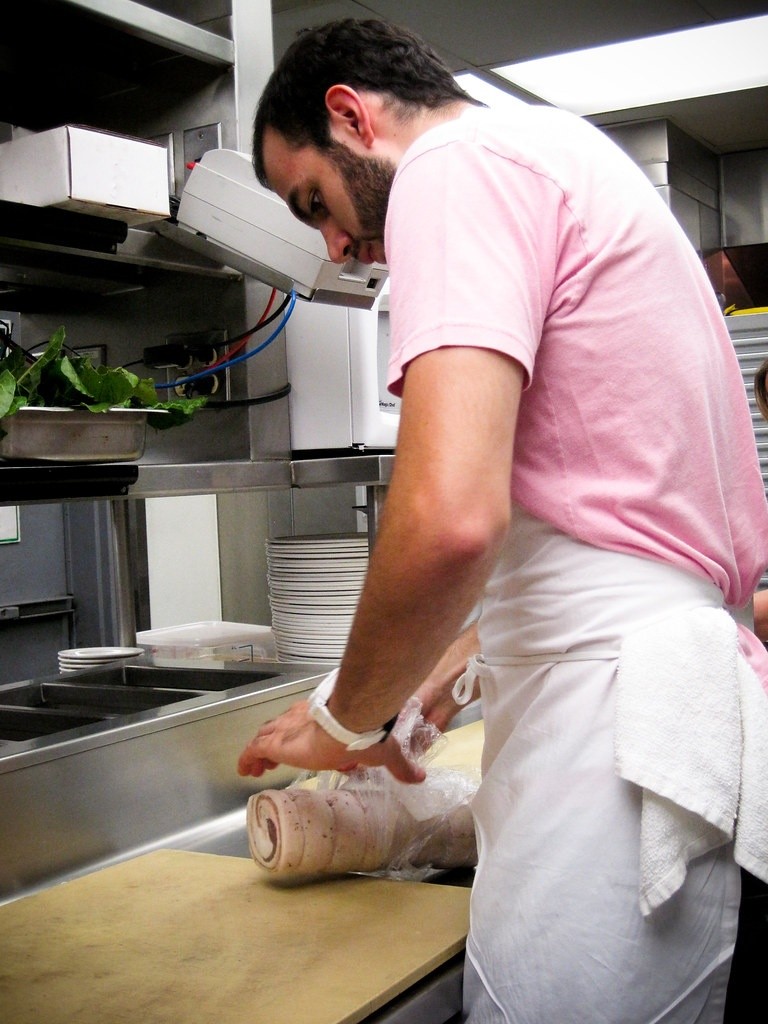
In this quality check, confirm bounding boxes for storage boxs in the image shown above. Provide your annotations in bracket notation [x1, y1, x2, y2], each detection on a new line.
[0, 124, 171, 228]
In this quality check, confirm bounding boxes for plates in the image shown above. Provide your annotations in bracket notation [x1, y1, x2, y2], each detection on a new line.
[57, 647, 145, 673]
[264, 532, 369, 665]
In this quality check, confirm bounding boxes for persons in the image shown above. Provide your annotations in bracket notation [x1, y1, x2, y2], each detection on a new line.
[234, 14, 766, 1024]
[750, 369, 766, 650]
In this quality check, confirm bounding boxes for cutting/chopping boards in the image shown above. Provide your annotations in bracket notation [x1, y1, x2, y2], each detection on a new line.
[0, 847, 473, 1024]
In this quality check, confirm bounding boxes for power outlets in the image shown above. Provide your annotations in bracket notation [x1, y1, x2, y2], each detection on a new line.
[166, 329, 230, 413]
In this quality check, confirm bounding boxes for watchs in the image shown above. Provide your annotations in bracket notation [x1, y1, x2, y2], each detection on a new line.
[305, 666, 402, 752]
